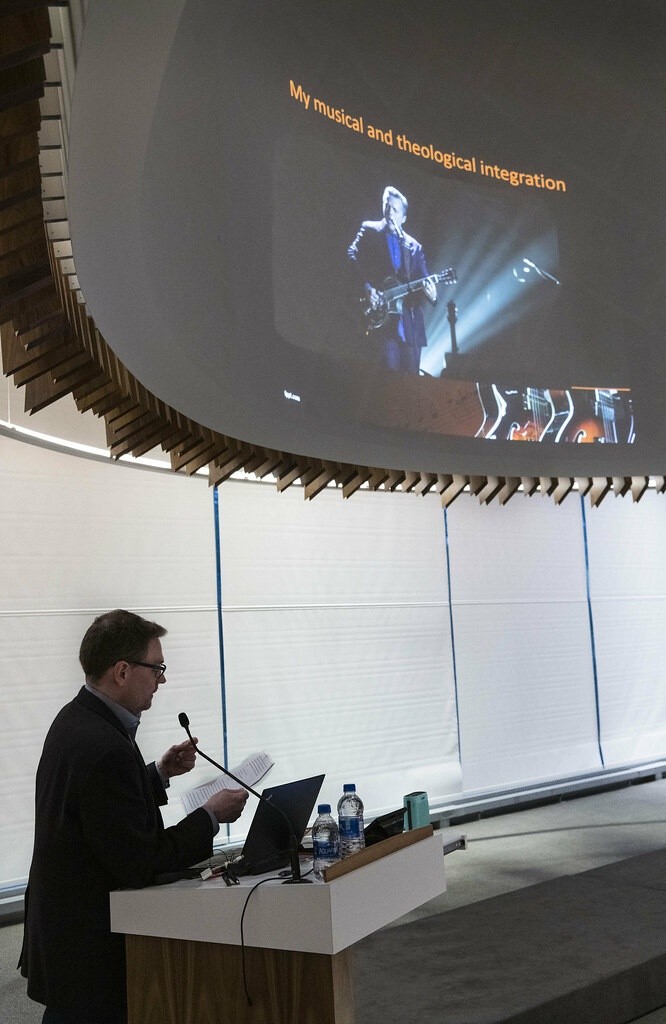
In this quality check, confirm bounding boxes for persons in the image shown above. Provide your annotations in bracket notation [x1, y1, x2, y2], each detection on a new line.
[345, 186, 438, 375]
[16, 609, 249, 1024]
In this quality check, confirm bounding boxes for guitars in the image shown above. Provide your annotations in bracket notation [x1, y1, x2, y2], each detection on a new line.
[359, 264, 461, 330]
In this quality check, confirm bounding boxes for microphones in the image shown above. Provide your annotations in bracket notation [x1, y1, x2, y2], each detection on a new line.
[178, 712, 314, 885]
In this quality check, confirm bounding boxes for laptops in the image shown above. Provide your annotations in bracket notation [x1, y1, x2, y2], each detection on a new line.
[182, 773, 326, 879]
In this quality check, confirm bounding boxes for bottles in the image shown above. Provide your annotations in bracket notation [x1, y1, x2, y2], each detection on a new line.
[312, 804, 338, 879]
[337, 784, 365, 860]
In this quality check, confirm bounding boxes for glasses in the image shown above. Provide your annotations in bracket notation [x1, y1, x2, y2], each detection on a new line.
[137, 662, 166, 678]
